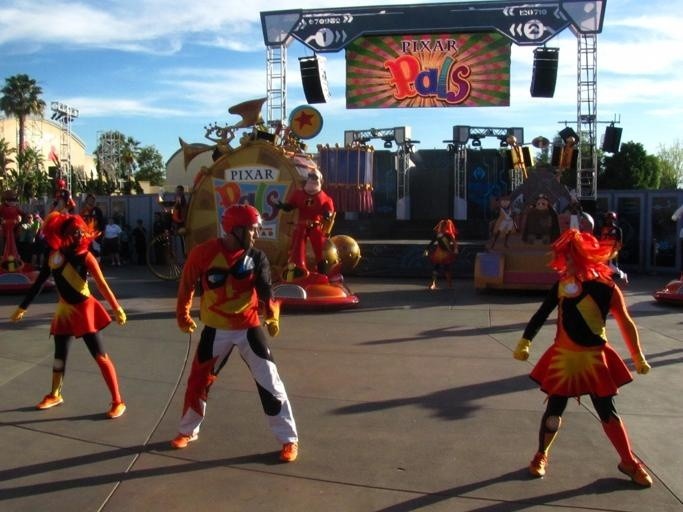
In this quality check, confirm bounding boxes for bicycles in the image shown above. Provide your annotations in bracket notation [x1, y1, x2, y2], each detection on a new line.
[145, 231, 187, 281]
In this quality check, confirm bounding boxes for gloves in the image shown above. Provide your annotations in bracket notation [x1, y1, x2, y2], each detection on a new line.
[631, 350, 652, 376]
[113, 306, 127, 327]
[514, 336, 533, 363]
[12, 307, 27, 324]
[263, 300, 282, 338]
[177, 282, 197, 334]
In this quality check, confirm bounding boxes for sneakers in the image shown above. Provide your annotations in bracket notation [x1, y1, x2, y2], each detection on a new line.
[107, 402, 127, 419]
[618, 459, 654, 489]
[170, 432, 199, 450]
[36, 394, 63, 410]
[281, 439, 299, 463]
[529, 452, 547, 478]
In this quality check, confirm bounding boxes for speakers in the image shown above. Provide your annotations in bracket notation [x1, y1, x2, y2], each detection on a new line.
[559, 128, 579, 146]
[531, 51, 558, 97]
[300, 57, 332, 104]
[603, 127, 622, 153]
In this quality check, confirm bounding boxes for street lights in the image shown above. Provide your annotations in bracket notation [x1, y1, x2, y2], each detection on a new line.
[49, 101, 80, 196]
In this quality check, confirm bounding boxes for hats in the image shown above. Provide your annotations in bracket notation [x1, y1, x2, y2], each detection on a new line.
[220, 205, 264, 233]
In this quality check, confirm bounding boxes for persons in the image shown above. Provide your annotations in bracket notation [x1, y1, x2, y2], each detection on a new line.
[490, 194, 623, 269]
[514, 229, 653, 486]
[277, 168, 334, 278]
[14, 179, 187, 271]
[421, 218, 459, 291]
[11, 212, 126, 419]
[170, 205, 299, 462]
